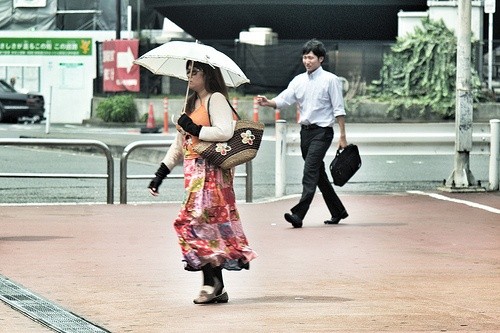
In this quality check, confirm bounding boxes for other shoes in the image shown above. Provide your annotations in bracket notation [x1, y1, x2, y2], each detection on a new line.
[284, 212, 304, 229]
[323, 209, 349, 224]
[193, 285, 230, 305]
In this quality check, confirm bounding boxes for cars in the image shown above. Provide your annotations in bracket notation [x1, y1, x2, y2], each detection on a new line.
[0, 79, 46, 124]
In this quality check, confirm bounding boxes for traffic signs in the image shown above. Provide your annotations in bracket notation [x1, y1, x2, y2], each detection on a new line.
[102, 40, 140, 93]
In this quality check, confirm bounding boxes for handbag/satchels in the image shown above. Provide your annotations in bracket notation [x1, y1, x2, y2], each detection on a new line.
[328, 144, 363, 187]
[192, 91, 266, 169]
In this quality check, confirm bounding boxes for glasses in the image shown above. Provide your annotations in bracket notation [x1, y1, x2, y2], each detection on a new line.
[186, 68, 204, 76]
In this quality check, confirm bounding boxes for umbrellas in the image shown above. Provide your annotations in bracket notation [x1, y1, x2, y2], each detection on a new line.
[132, 40, 250, 125]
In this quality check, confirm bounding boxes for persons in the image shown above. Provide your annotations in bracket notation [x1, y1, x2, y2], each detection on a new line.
[257, 39, 349, 229]
[9, 77, 15, 88]
[146, 60, 256, 305]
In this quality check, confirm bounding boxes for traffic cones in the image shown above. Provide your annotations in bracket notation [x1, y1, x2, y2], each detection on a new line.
[140, 104, 159, 134]
